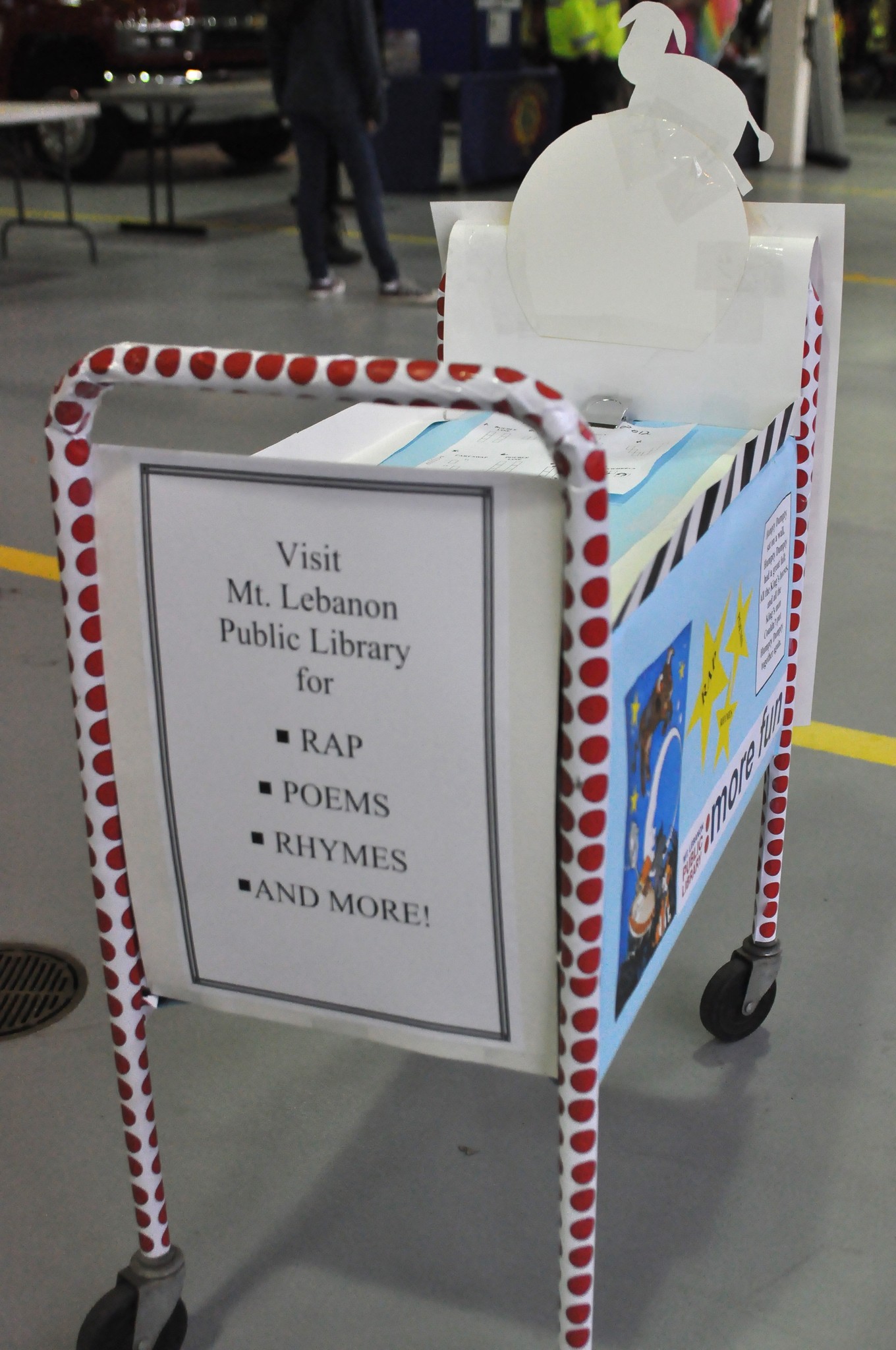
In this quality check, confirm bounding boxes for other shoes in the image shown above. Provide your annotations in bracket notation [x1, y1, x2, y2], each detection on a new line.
[307, 269, 347, 299]
[378, 279, 436, 303]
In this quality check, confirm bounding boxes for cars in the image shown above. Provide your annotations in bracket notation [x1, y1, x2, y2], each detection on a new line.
[0, 1, 290, 182]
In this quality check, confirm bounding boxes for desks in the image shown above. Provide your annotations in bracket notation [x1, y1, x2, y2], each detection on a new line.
[0, 101, 101, 268]
[84, 75, 273, 235]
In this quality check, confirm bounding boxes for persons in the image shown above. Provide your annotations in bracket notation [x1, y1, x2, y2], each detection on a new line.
[718, 0, 753, 84]
[374, 0, 477, 192]
[867, 0, 889, 60]
[266, 0, 434, 302]
[545, 0, 627, 134]
[660, 0, 696, 55]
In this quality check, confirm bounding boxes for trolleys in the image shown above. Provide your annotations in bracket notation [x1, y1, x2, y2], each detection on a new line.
[44, 263, 826, 1349]
